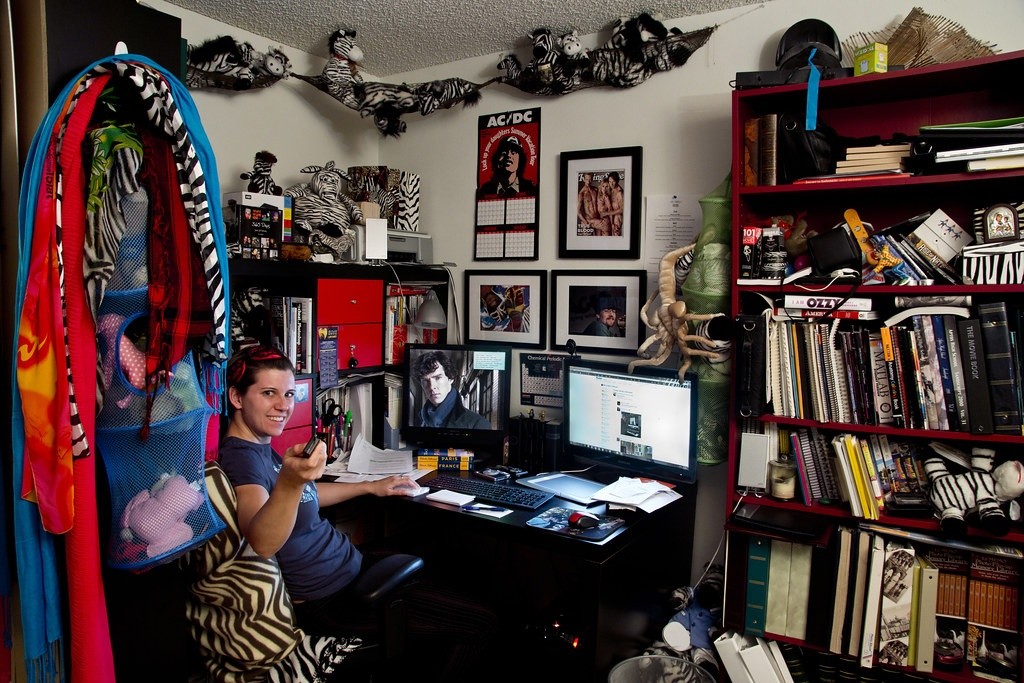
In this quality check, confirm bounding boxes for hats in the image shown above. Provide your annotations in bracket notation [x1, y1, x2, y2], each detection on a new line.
[591, 290, 615, 313]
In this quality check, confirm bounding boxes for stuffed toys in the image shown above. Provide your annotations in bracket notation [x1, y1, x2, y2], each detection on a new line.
[187, 11, 716, 137]
[920, 440, 1024, 540]
[240, 151, 364, 238]
[637, 561, 728, 683]
[122, 471, 206, 557]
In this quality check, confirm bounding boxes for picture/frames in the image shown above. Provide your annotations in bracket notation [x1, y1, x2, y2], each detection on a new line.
[550, 269, 648, 357]
[559, 146, 643, 260]
[464, 270, 548, 350]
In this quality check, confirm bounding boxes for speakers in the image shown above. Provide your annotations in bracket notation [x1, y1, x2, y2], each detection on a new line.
[738, 434, 770, 488]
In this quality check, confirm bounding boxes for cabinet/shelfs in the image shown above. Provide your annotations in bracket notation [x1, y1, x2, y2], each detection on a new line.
[227, 258, 451, 464]
[726, 49, 1024, 683]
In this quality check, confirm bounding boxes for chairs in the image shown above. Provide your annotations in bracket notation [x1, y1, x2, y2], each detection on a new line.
[182, 460, 423, 683]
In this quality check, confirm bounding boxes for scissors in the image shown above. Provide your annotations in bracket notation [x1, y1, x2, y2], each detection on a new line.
[322, 399, 343, 428]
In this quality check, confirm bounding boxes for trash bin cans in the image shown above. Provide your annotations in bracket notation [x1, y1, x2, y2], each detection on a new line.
[607, 655, 716, 683]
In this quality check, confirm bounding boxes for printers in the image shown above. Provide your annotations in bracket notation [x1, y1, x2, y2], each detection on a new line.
[339, 224, 458, 269]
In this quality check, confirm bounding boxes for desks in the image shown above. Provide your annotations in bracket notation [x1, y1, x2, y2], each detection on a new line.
[315, 456, 699, 683]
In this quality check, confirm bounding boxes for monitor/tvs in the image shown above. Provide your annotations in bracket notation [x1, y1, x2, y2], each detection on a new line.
[402, 343, 513, 471]
[562, 358, 699, 485]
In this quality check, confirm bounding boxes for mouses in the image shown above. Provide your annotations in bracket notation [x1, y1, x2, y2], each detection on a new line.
[567, 512, 599, 529]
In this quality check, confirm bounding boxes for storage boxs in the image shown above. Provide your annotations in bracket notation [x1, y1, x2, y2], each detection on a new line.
[223, 191, 292, 258]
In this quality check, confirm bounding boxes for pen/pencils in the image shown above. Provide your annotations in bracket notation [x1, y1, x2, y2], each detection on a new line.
[317, 419, 322, 433]
[323, 427, 331, 433]
[335, 410, 353, 453]
[329, 423, 336, 459]
[462, 506, 507, 512]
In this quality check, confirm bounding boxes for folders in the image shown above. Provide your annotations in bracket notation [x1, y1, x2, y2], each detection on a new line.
[713, 632, 753, 683]
[738, 634, 785, 683]
[915, 553, 939, 674]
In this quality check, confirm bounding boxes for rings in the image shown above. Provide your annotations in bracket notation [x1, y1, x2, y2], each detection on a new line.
[400, 476, 403, 478]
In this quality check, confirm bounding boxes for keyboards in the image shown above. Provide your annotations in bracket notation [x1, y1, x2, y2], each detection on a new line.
[423, 476, 557, 512]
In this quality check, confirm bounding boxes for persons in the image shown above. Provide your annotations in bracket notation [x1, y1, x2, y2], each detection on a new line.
[582, 297, 621, 337]
[413, 351, 493, 430]
[218, 345, 420, 631]
[577, 171, 625, 238]
[863, 241, 912, 285]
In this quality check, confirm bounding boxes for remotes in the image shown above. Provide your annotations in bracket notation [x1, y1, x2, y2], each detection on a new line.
[303, 435, 319, 457]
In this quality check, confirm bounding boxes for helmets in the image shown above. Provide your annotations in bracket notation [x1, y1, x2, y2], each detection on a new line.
[775, 18, 842, 67]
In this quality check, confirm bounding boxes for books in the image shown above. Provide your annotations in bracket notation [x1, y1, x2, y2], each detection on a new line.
[384, 281, 439, 364]
[417, 448, 475, 471]
[739, 110, 1024, 683]
[266, 296, 313, 376]
